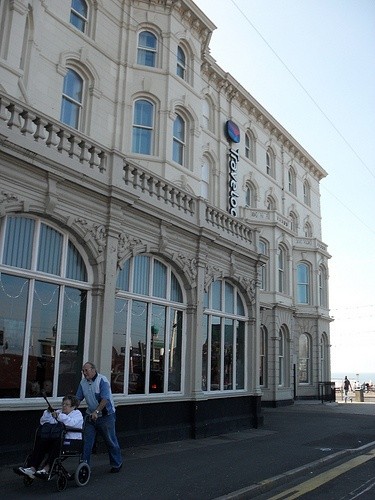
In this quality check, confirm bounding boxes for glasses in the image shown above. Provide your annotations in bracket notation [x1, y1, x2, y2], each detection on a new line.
[81, 368, 92, 373]
[62, 403, 70, 406]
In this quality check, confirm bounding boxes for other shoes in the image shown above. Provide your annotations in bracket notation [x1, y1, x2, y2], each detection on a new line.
[13, 466, 36, 480]
[36, 468, 49, 475]
[109, 464, 122, 473]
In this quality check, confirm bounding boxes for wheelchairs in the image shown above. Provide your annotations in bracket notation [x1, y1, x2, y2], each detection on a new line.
[13, 414, 93, 492]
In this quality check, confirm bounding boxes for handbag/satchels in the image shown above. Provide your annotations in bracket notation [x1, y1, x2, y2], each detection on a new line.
[41, 422, 66, 438]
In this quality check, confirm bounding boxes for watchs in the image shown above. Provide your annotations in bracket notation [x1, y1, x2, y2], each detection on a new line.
[96, 410, 100, 415]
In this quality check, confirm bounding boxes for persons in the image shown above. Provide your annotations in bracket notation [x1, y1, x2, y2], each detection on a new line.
[73, 361, 122, 474]
[36, 380, 52, 396]
[343, 376, 352, 402]
[19, 395, 83, 481]
